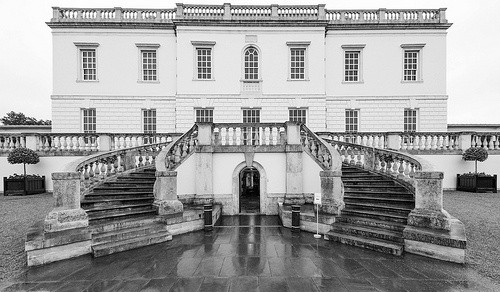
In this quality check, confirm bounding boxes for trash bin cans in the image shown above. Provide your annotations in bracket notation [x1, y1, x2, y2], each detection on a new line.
[291, 205, 301, 232]
[203, 205, 214, 232]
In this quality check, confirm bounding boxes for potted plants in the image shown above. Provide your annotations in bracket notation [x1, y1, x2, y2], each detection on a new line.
[3, 147, 47, 197]
[454, 146, 498, 194]
